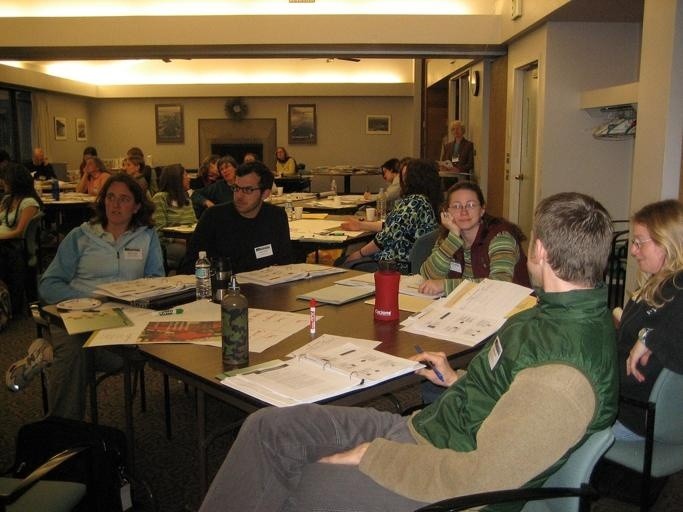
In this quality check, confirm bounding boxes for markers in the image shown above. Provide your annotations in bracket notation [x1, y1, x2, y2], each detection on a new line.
[148, 308, 185, 316]
[309, 299, 317, 335]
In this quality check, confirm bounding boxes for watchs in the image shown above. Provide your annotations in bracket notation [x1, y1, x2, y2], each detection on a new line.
[637, 328, 654, 345]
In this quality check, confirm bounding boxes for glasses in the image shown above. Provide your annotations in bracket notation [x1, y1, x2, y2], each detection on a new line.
[231, 183, 265, 194]
[631, 238, 653, 250]
[452, 202, 479, 210]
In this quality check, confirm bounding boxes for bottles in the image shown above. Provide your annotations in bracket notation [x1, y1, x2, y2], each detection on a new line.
[195, 250, 210, 304]
[285, 202, 292, 222]
[212, 243, 232, 304]
[372, 258, 401, 320]
[377, 187, 386, 222]
[222, 278, 249, 366]
[331, 179, 337, 200]
[53, 179, 59, 200]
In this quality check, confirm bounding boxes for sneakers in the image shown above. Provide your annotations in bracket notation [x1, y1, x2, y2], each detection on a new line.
[5, 338, 53, 392]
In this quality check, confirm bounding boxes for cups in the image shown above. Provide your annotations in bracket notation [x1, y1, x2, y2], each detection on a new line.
[294, 207, 303, 219]
[278, 187, 284, 196]
[366, 208, 377, 222]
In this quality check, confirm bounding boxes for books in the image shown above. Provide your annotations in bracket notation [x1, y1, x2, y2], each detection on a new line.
[399, 277, 535, 349]
[237, 258, 350, 287]
[214, 343, 427, 407]
[91, 272, 201, 302]
[297, 268, 446, 306]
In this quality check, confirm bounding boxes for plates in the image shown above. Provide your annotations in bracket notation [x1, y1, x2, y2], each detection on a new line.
[56, 298, 102, 310]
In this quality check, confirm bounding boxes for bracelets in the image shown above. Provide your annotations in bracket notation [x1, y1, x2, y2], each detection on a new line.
[360, 246, 364, 257]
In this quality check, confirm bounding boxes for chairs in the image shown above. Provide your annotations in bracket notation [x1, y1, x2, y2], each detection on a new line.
[591, 367, 683, 512]
[604, 219, 630, 310]
[414, 426, 616, 512]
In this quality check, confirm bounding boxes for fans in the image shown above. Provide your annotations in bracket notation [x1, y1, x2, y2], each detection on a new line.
[223, 96, 252, 121]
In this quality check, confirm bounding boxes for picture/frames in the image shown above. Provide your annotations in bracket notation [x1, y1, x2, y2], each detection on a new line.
[75, 117, 87, 141]
[365, 115, 391, 134]
[55, 117, 68, 140]
[288, 103, 316, 145]
[155, 102, 184, 144]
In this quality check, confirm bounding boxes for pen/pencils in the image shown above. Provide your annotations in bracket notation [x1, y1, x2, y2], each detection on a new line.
[409, 284, 419, 290]
[415, 345, 445, 383]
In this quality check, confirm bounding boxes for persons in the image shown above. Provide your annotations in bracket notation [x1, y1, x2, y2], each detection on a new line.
[334, 151, 446, 280]
[611, 198, 683, 442]
[199, 191, 619, 512]
[417, 180, 536, 296]
[1, 143, 299, 289]
[6, 172, 167, 418]
[440, 121, 474, 187]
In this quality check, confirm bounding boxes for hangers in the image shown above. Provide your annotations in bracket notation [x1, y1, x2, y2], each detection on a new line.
[593, 103, 638, 142]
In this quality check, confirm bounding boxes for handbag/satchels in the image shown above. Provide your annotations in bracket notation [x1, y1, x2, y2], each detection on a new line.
[12, 417, 158, 512]
